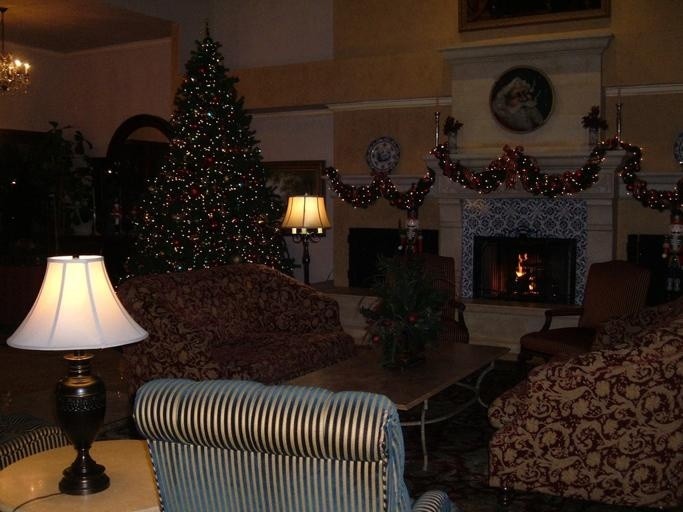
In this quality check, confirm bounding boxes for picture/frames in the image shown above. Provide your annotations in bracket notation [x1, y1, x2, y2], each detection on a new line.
[258, 158, 327, 224]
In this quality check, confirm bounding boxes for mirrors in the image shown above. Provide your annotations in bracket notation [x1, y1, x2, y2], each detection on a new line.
[95, 111, 177, 287]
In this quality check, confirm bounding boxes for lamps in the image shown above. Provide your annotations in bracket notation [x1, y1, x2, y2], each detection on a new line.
[0, 8, 33, 99]
[280, 191, 332, 284]
[5, 241, 149, 494]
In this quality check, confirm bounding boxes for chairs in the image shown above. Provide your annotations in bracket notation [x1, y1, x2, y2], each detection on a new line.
[386, 252, 470, 344]
[518, 260, 653, 369]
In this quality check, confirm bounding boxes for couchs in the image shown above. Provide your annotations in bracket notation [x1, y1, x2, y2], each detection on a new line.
[0, 410, 73, 470]
[116, 263, 358, 392]
[132, 379, 460, 512]
[486, 294, 683, 506]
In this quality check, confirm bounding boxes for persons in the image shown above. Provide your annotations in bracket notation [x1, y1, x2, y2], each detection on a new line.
[492, 75, 545, 131]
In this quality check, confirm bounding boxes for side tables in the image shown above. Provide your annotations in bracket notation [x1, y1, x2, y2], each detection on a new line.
[0, 439, 162, 512]
